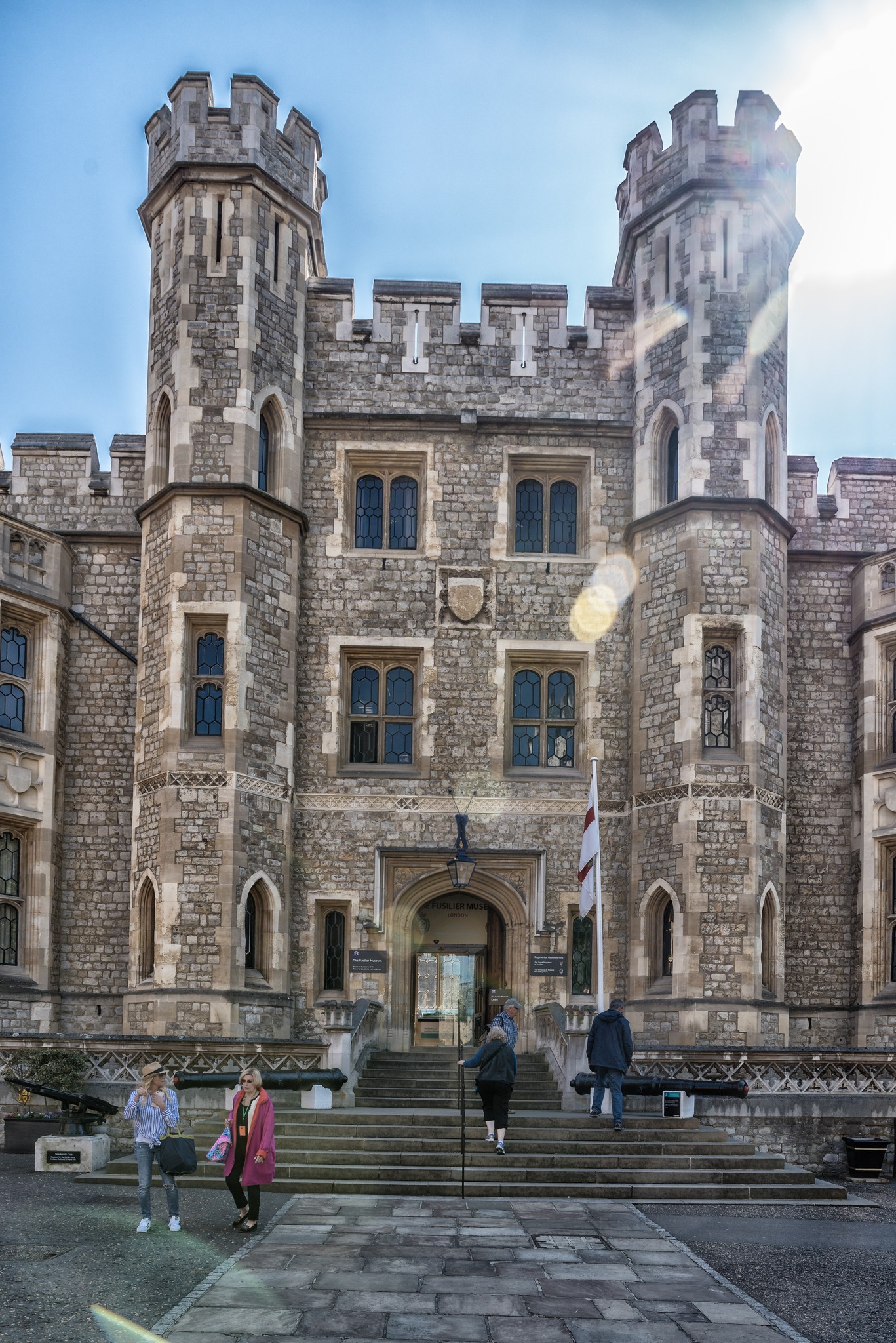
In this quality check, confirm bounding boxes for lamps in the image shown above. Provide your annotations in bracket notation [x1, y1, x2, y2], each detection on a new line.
[441, 787, 480, 891]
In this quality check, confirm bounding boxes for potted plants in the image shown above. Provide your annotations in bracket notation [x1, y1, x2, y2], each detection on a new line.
[0, 1046, 91, 1154]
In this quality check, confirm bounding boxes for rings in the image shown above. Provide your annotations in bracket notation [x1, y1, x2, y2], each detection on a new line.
[262, 1161, 263, 1162]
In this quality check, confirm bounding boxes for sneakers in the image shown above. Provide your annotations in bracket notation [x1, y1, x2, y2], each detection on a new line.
[614, 1122, 624, 1130]
[496, 1142, 505, 1155]
[589, 1110, 598, 1118]
[137, 1217, 151, 1232]
[485, 1133, 495, 1142]
[168, 1216, 181, 1231]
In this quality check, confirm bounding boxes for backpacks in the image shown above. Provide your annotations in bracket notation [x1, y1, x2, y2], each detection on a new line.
[479, 1015, 504, 1049]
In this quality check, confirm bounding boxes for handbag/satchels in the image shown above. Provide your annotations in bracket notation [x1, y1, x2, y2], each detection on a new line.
[159, 1118, 197, 1176]
[205, 1108, 234, 1164]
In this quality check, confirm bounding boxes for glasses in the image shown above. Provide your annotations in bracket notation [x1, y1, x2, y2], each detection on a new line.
[511, 1007, 519, 1010]
[621, 1008, 624, 1014]
[155, 1072, 166, 1078]
[242, 1079, 253, 1084]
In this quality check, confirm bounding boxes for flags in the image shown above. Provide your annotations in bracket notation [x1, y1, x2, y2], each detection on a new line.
[577, 770, 601, 922]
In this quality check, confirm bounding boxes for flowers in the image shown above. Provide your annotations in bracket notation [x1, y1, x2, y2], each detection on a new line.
[16, 1110, 62, 1119]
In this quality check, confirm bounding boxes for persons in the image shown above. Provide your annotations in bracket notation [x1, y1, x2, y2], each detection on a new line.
[479, 998, 523, 1114]
[124, 1062, 180, 1232]
[586, 998, 633, 1130]
[457, 1027, 512, 1144]
[224, 1068, 274, 1233]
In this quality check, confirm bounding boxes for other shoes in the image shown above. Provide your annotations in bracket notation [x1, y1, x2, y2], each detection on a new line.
[508, 1109, 516, 1114]
[232, 1209, 249, 1227]
[239, 1221, 258, 1233]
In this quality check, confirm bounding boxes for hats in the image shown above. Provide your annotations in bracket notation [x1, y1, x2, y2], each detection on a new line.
[505, 998, 524, 1008]
[141, 1061, 170, 1081]
